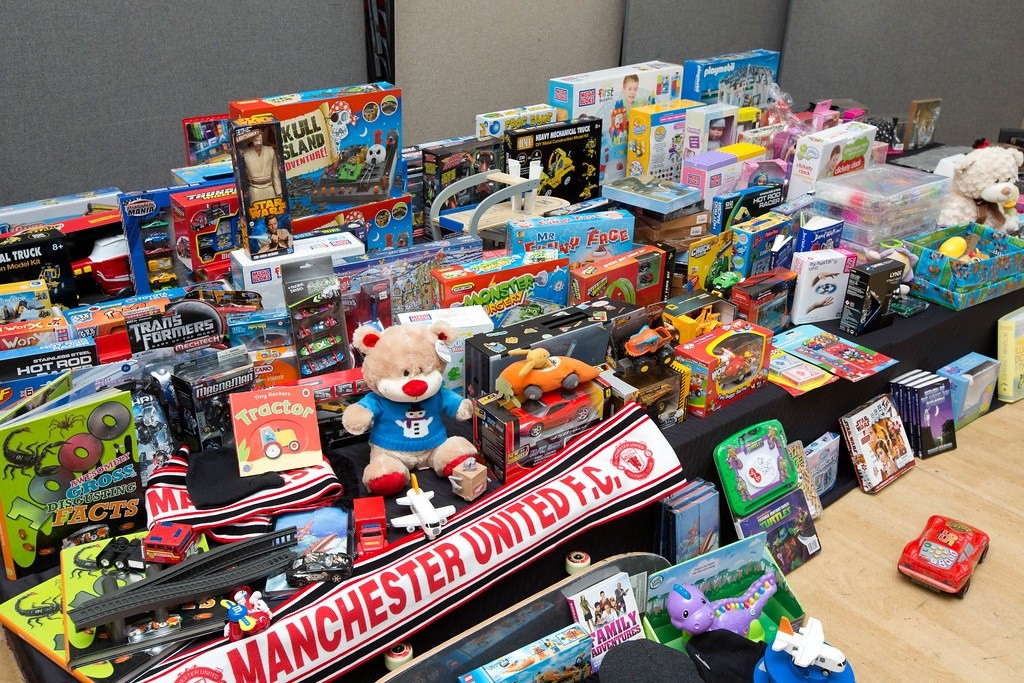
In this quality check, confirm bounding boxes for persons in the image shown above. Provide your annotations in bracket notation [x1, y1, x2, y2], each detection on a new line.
[580, 583, 628, 631]
[863, 425, 887, 453]
[873, 440, 898, 481]
[781, 129, 804, 192]
[615, 75, 646, 121]
[709, 116, 725, 152]
[820, 147, 841, 176]
[237, 129, 282, 209]
[256, 215, 293, 256]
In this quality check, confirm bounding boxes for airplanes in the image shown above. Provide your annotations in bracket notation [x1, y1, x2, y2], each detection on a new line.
[770, 615, 846, 677]
[389, 473, 456, 543]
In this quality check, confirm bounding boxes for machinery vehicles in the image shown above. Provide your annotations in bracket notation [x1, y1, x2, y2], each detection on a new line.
[0, 300, 46, 326]
[536, 148, 575, 196]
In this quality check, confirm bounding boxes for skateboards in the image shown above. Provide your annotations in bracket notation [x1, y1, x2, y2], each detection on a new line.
[300, 333, 343, 356]
[292, 303, 332, 320]
[300, 351, 346, 375]
[295, 316, 341, 339]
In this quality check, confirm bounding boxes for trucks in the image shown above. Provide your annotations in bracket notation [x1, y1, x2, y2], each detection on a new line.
[352, 496, 390, 561]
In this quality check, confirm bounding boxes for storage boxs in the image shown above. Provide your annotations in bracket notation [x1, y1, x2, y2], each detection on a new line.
[1, 43, 1023, 683]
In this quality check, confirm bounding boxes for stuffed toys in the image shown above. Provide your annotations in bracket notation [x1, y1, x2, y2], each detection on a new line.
[341, 324, 481, 495]
[935, 146, 1024, 237]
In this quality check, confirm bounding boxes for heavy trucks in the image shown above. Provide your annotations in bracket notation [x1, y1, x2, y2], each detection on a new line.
[95, 522, 206, 574]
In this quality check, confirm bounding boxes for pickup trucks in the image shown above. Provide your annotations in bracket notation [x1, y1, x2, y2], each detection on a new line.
[618, 325, 675, 376]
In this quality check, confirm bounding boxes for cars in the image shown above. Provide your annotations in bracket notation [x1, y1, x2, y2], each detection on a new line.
[143, 230, 170, 246]
[145, 244, 174, 256]
[715, 346, 745, 388]
[151, 284, 178, 293]
[148, 257, 174, 272]
[285, 552, 354, 589]
[508, 387, 592, 437]
[149, 272, 178, 285]
[897, 515, 990, 600]
[140, 219, 169, 230]
[629, 375, 676, 414]
